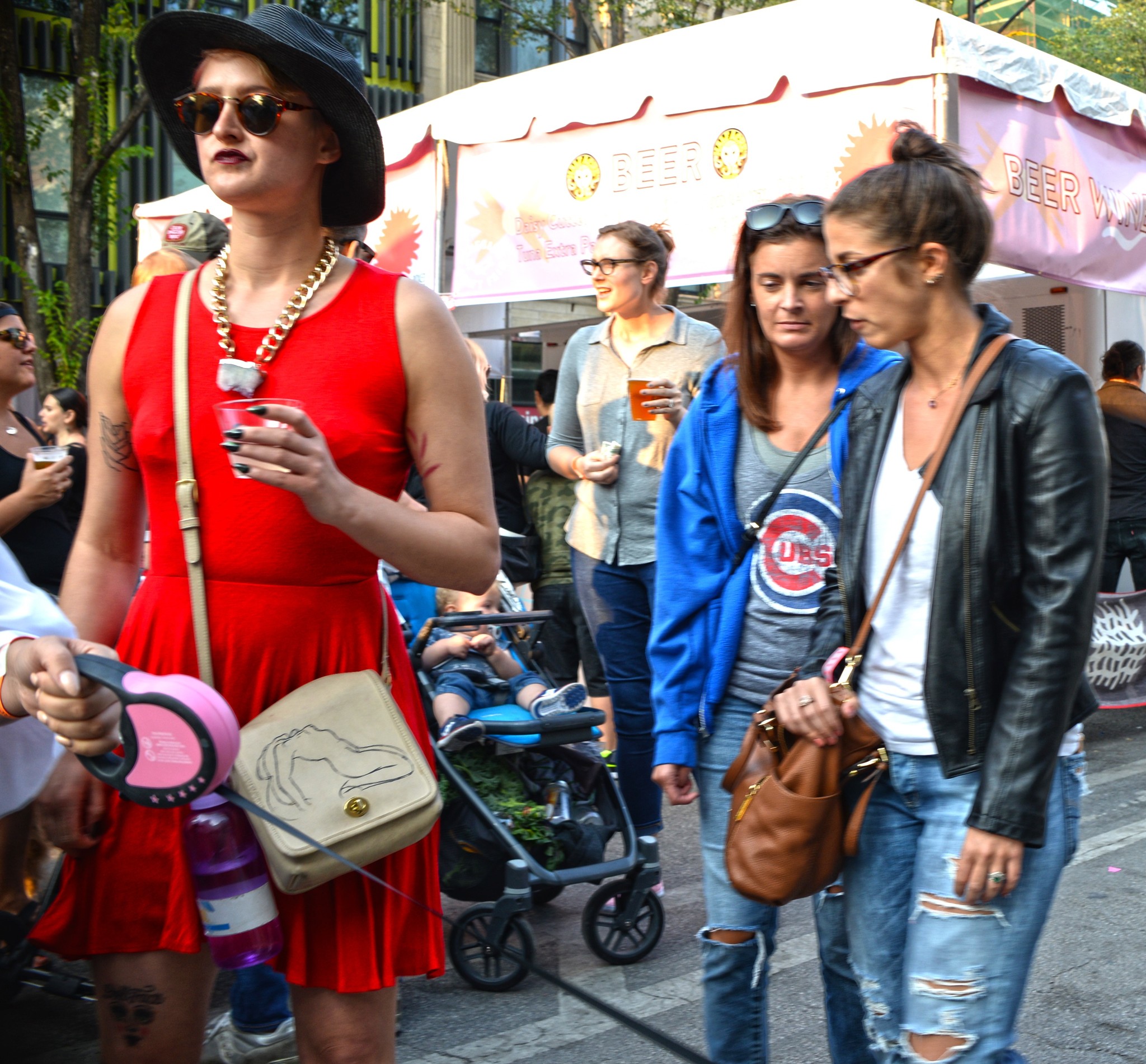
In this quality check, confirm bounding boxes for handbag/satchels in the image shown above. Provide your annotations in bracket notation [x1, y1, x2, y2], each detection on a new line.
[224, 668, 444, 896]
[723, 668, 887, 904]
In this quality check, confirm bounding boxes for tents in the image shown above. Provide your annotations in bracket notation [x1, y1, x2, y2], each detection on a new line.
[133, 0, 1146, 309]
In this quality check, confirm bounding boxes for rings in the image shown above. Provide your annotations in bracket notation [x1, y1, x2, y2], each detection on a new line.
[668, 399, 673, 408]
[987, 871, 1006, 884]
[797, 695, 813, 707]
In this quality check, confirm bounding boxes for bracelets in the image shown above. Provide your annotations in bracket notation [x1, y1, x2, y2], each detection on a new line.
[572, 455, 587, 479]
[0, 635, 39, 720]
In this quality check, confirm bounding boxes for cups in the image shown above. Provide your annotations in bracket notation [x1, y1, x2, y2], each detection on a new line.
[30, 445, 71, 470]
[627, 378, 658, 421]
[215, 397, 304, 479]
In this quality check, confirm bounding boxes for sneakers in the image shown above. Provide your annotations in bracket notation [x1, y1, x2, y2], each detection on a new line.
[530, 683, 586, 719]
[199, 1009, 303, 1064]
[437, 714, 485, 756]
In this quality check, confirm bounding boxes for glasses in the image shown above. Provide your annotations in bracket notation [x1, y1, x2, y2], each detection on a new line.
[340, 238, 375, 264]
[818, 246, 961, 296]
[485, 364, 492, 377]
[0, 328, 36, 350]
[746, 201, 826, 231]
[581, 259, 646, 276]
[172, 91, 318, 136]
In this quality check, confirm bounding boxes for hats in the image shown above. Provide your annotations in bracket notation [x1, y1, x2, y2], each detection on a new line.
[164, 211, 229, 262]
[136, 3, 385, 227]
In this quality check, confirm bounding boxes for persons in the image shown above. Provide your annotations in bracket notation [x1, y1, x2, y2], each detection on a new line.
[0, 0, 1146, 1064]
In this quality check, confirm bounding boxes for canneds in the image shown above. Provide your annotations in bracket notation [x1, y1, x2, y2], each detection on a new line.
[571, 806, 604, 826]
[542, 781, 571, 825]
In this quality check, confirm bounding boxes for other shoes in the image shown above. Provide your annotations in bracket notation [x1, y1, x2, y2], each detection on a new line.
[601, 879, 665, 913]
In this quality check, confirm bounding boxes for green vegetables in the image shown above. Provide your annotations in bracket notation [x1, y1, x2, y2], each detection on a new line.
[440, 751, 565, 887]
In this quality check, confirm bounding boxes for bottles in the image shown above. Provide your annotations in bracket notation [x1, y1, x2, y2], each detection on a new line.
[542, 781, 572, 824]
[179, 794, 285, 973]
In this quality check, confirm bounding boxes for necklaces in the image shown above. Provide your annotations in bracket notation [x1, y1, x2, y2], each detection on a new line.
[5, 425, 18, 435]
[212, 238, 339, 393]
[924, 369, 961, 410]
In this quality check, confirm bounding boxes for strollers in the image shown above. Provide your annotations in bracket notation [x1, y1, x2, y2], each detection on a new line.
[370, 560, 668, 990]
